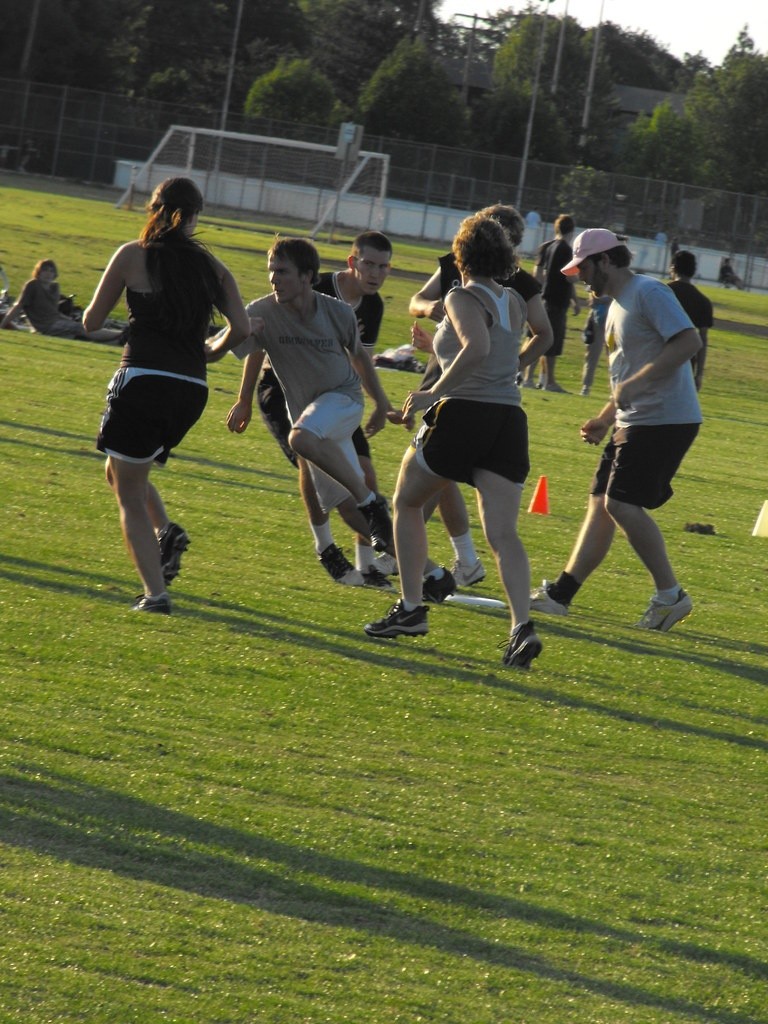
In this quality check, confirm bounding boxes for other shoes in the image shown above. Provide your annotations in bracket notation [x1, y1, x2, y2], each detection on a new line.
[516, 373, 537, 389]
[536, 380, 567, 393]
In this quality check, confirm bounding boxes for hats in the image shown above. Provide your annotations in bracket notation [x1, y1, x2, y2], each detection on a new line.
[560, 229, 627, 276]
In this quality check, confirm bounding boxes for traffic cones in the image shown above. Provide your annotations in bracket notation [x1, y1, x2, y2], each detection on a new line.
[529, 476, 551, 516]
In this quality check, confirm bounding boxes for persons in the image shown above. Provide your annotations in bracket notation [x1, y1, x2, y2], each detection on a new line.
[206, 232, 458, 605]
[525, 228, 707, 628]
[0, 132, 35, 172]
[364, 218, 552, 669]
[83, 177, 250, 614]
[717, 259, 742, 289]
[654, 226, 714, 392]
[373, 204, 630, 586]
[0, 260, 130, 342]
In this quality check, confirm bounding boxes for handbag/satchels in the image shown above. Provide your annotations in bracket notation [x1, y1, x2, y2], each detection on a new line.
[584, 309, 594, 344]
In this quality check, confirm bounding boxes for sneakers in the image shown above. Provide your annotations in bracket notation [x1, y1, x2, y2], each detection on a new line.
[131, 592, 171, 617]
[496, 620, 542, 669]
[156, 522, 192, 586]
[373, 552, 398, 576]
[316, 543, 365, 586]
[631, 588, 693, 631]
[451, 556, 487, 586]
[364, 599, 430, 638]
[530, 578, 570, 616]
[420, 566, 456, 603]
[357, 565, 397, 593]
[360, 492, 392, 553]
